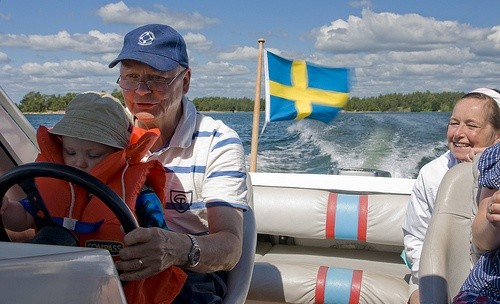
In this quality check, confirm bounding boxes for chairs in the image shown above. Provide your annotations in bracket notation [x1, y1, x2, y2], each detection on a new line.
[213, 173, 257, 304]
[419, 154, 480, 304]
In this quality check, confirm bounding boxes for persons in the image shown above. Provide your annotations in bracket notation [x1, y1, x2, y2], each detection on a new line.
[399, 88, 500, 304]
[0, 90, 189, 304]
[5, 23, 248, 304]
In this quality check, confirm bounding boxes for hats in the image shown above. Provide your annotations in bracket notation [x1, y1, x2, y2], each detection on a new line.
[108, 24, 189, 71]
[48, 91, 134, 149]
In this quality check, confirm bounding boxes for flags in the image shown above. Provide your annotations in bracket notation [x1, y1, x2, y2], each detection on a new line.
[263, 48, 357, 126]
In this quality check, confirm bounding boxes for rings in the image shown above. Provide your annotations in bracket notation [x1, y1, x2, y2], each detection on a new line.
[139, 258, 145, 270]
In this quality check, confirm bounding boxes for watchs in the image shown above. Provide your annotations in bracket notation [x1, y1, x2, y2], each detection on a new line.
[182, 234, 201, 270]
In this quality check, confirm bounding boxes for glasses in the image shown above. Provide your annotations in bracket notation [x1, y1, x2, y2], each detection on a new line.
[115, 67, 189, 95]
